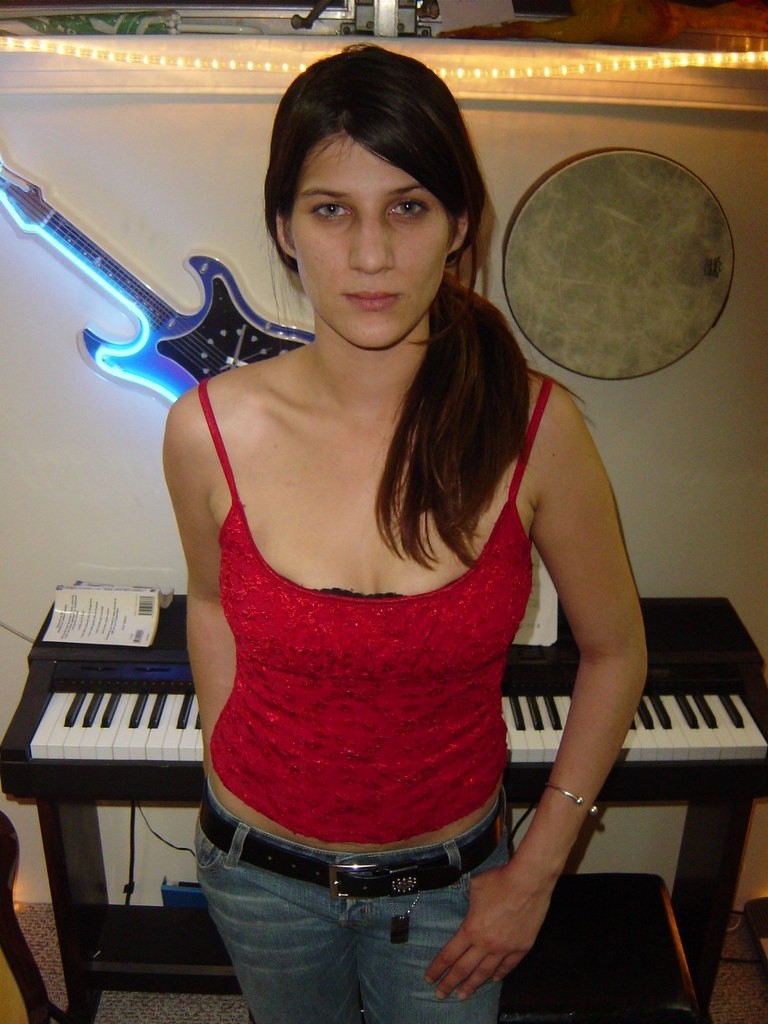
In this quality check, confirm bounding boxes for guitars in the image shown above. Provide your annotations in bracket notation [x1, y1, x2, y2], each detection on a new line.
[0, 155, 315, 405]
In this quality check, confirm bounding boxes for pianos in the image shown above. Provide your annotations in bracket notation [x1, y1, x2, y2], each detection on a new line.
[0, 581, 768, 1024]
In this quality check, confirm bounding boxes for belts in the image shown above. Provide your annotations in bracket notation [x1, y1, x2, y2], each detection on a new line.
[199, 777, 506, 897]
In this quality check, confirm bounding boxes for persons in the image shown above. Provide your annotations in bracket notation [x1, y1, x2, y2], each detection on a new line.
[161, 42, 649, 1024]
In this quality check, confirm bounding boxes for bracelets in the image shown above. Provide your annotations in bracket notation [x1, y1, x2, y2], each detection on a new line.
[543, 780, 601, 817]
